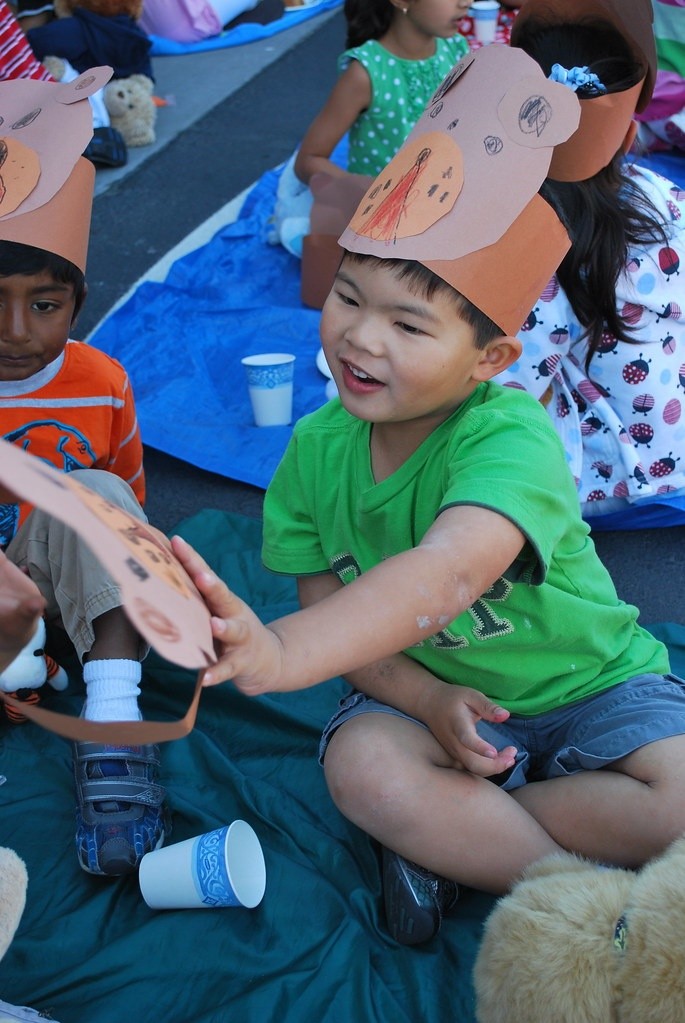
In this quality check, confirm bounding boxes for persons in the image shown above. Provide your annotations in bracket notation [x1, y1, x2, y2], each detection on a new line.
[172, 43, 685, 896]
[0, 65, 168, 872]
[0, 0, 267, 84]
[292, 0, 685, 503]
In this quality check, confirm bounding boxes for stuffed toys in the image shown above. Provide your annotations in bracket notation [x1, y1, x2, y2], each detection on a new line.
[470, 832, 685, 1023]
[41, 55, 157, 148]
[0, 846, 29, 961]
[53, 0, 143, 22]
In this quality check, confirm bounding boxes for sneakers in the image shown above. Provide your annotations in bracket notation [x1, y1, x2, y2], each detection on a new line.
[382, 842, 462, 948]
[72, 700, 173, 877]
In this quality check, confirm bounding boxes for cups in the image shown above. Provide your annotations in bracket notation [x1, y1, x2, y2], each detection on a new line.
[139, 820, 266, 910]
[241, 353, 296, 426]
[471, 0, 501, 43]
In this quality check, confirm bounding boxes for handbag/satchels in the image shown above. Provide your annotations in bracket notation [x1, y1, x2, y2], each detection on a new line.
[25, 5, 156, 86]
